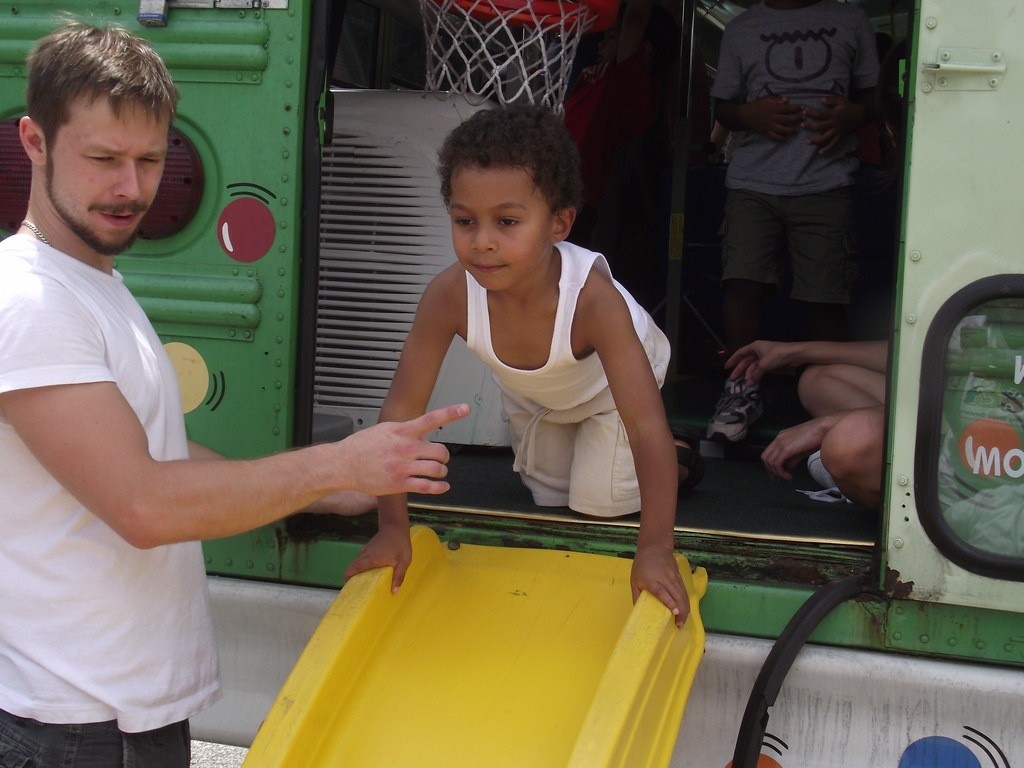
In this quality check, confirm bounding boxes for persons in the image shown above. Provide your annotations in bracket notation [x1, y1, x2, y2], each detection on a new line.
[702, 0, 907, 445]
[724, 339, 887, 514]
[343, 102, 706, 631]
[562, 0, 677, 250]
[0, 10, 470, 768]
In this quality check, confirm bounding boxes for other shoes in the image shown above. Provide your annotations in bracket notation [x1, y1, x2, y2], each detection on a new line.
[795, 450, 852, 504]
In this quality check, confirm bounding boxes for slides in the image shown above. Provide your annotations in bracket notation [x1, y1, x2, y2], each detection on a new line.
[245, 524, 708, 767]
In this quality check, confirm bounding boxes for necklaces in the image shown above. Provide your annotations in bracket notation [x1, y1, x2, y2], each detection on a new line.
[21, 221, 49, 245]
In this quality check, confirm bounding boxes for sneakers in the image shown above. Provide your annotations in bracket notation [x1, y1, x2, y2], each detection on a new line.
[705, 374, 763, 443]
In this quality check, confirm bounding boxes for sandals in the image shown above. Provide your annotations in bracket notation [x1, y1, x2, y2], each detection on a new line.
[668, 421, 703, 490]
